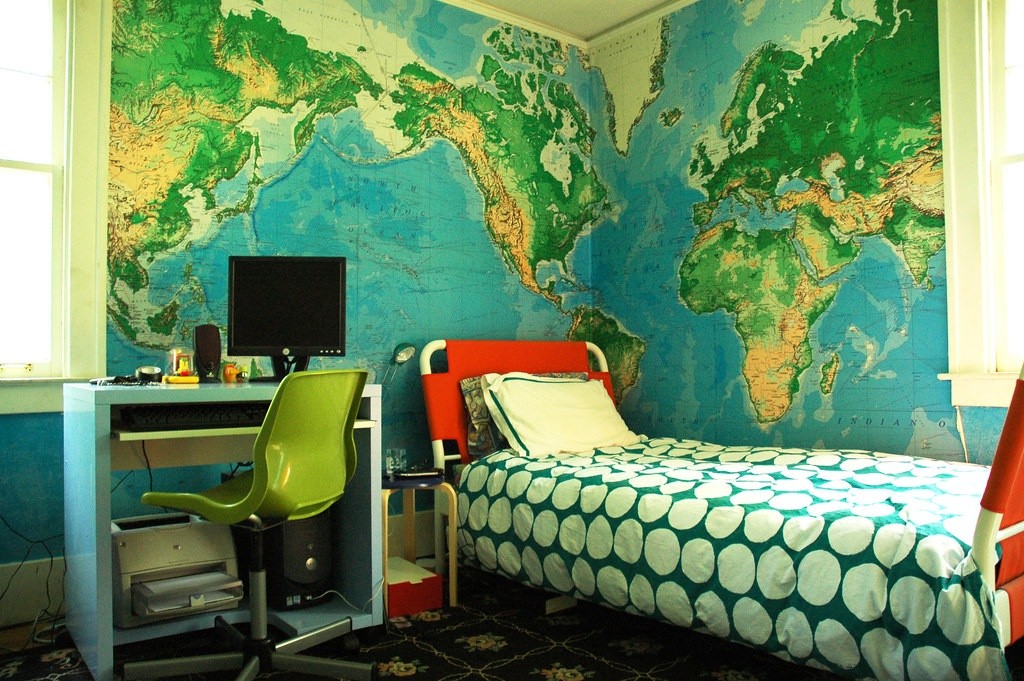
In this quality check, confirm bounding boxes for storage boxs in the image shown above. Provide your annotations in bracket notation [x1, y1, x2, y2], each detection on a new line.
[387, 556, 443, 617]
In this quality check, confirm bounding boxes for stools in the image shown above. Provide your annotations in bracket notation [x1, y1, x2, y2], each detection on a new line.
[382, 467, 458, 619]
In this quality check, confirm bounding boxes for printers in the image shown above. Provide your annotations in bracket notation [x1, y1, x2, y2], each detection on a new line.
[111, 512, 246, 628]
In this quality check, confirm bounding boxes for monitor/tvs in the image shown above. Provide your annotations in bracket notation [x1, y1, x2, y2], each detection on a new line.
[227, 256, 346, 382]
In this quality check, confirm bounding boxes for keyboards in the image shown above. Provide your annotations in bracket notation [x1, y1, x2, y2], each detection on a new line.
[120, 402, 272, 432]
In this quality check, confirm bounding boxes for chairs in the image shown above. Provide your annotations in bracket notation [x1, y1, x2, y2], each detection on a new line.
[119, 368, 380, 681]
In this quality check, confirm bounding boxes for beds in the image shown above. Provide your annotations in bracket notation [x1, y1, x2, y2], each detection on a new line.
[419, 336, 1024, 680]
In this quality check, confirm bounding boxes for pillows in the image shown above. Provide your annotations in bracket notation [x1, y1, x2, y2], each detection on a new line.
[480, 371, 643, 457]
[459, 371, 588, 461]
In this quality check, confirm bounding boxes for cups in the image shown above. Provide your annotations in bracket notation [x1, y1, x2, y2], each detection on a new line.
[386, 449, 407, 473]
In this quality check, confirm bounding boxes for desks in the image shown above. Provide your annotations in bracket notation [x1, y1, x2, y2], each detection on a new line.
[62, 381, 383, 681]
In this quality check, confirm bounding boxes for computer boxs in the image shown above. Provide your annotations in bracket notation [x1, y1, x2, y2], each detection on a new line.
[223, 471, 336, 611]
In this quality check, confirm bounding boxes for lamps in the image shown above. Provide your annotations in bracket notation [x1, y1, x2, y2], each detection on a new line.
[381, 342, 416, 401]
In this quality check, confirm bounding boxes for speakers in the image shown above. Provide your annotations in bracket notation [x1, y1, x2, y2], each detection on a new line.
[193, 324, 222, 383]
